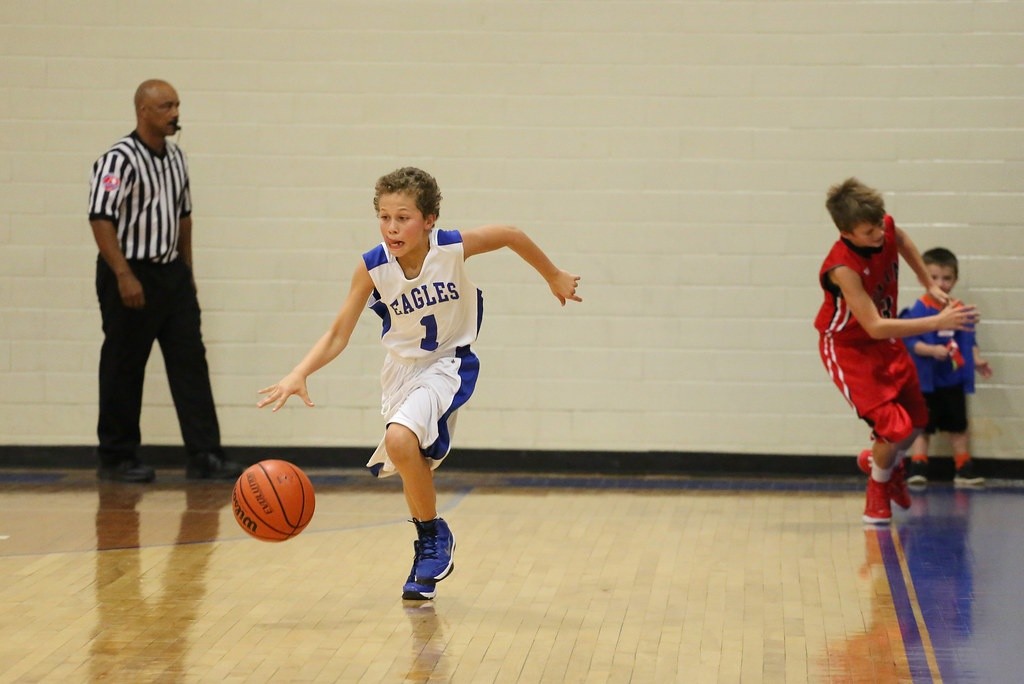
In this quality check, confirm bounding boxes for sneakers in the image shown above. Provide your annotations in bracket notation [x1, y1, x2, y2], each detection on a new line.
[858, 450, 912, 511]
[408, 517, 456, 584]
[402, 540, 437, 600]
[863, 474, 893, 524]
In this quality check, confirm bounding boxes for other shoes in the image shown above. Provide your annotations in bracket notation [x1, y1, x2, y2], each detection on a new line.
[96, 460, 155, 481]
[906, 460, 929, 486]
[954, 460, 985, 485]
[187, 458, 241, 481]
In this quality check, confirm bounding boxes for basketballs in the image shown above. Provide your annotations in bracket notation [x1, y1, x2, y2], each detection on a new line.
[230, 458, 316, 543]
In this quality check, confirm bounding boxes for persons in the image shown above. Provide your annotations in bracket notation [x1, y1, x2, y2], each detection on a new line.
[256, 167, 582, 606]
[87, 78, 246, 484]
[814, 176, 993, 524]
